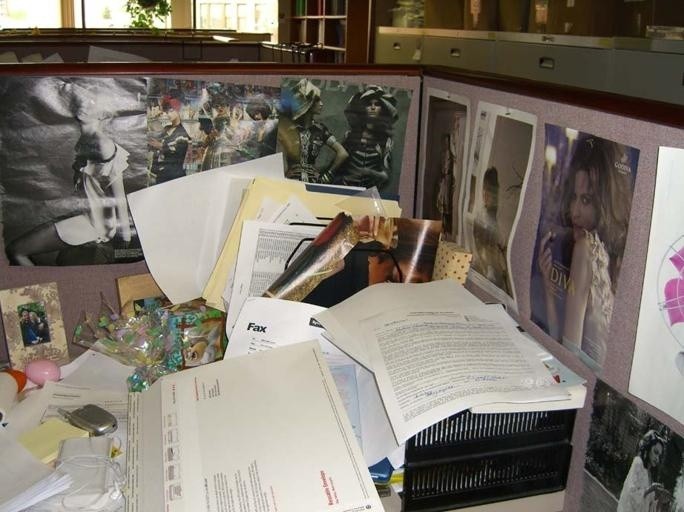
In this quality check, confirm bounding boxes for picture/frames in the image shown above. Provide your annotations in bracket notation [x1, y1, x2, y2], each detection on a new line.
[0, 282, 69, 377]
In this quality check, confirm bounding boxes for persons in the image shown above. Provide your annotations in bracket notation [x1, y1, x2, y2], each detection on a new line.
[335, 84, 400, 192]
[146, 90, 279, 187]
[616, 430, 670, 512]
[469, 165, 506, 288]
[19, 310, 50, 344]
[536, 136, 634, 368]
[4, 78, 132, 266]
[277, 78, 350, 186]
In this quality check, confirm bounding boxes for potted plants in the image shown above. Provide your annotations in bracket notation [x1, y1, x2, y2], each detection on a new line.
[126, 0, 171, 30]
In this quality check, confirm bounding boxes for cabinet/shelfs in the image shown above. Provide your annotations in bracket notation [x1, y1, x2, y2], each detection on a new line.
[290, 1, 370, 65]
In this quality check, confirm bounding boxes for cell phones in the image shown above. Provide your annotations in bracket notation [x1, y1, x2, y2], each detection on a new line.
[56, 404, 118, 437]
